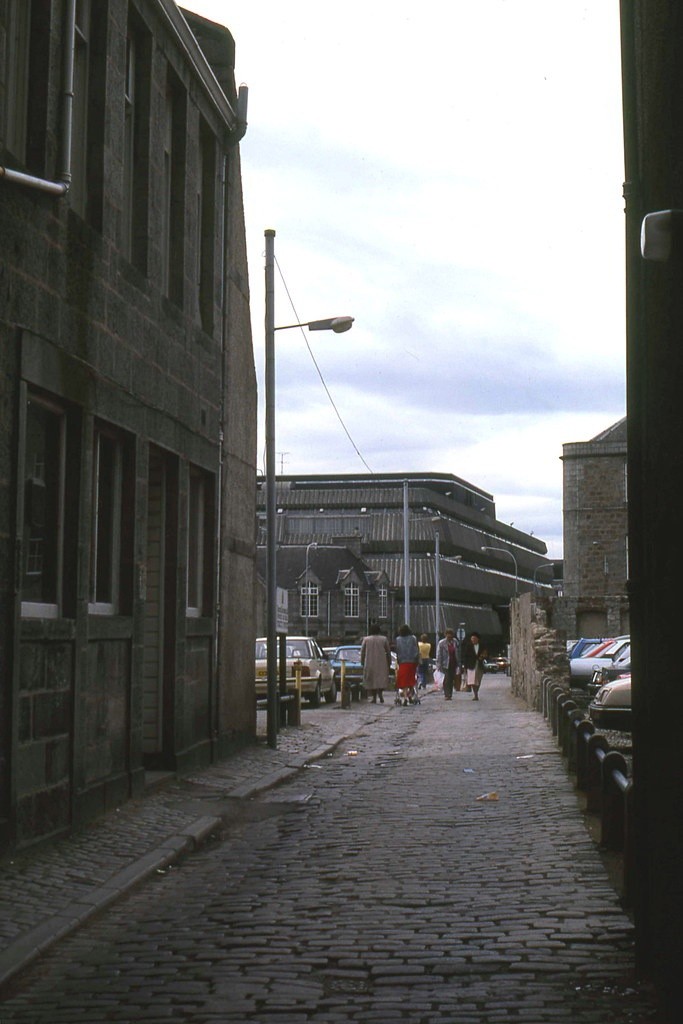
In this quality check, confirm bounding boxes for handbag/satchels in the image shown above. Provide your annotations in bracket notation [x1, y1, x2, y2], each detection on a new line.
[433, 668, 445, 691]
[467, 670, 479, 686]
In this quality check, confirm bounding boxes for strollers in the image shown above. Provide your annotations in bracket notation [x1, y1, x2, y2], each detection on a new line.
[394, 659, 420, 705]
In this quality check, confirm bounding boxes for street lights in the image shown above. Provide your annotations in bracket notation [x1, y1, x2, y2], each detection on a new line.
[480, 544, 519, 599]
[305, 541, 319, 637]
[258, 228, 356, 750]
[533, 562, 554, 594]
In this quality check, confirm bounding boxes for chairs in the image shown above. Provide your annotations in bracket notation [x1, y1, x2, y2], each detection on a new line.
[291, 648, 304, 658]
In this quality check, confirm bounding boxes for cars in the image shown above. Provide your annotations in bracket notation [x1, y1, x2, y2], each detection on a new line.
[484, 657, 511, 675]
[255, 635, 436, 708]
[565, 634, 632, 732]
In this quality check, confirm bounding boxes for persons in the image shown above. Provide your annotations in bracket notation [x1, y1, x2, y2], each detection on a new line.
[436, 628, 462, 700]
[361, 624, 391, 704]
[395, 624, 420, 707]
[418, 633, 431, 689]
[465, 632, 488, 701]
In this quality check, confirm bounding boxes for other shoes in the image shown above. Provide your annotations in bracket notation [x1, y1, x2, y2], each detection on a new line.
[472, 698, 478, 701]
[418, 682, 422, 689]
[445, 694, 452, 700]
[402, 699, 407, 706]
[370, 700, 376, 703]
[408, 697, 414, 704]
[380, 698, 384, 703]
